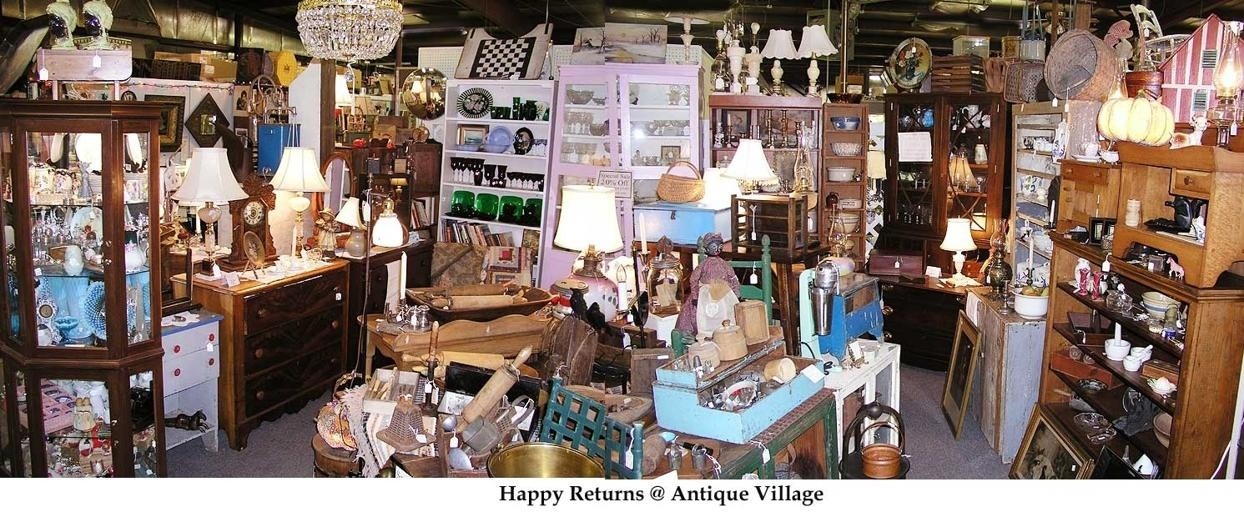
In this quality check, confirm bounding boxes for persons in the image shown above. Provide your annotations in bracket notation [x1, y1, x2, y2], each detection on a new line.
[675, 232, 741, 334]
[317, 208, 338, 262]
[237, 91, 247, 110]
[1030, 448, 1054, 479]
[74, 397, 95, 432]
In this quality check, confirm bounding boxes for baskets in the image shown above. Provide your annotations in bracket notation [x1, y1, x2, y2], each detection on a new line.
[856, 421, 905, 478]
[1003, 60, 1045, 104]
[735, 299, 770, 346]
[1044, 29, 1115, 99]
[657, 161, 706, 204]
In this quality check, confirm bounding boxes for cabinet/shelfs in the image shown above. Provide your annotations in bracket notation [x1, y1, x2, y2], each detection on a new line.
[0, 99, 163, 477]
[542, 67, 702, 290]
[161, 308, 226, 457]
[171, 257, 352, 453]
[1012, 111, 1069, 295]
[888, 95, 1000, 236]
[438, 78, 558, 287]
[1117, 153, 1224, 284]
[1042, 245, 1195, 477]
[1057, 160, 1118, 260]
[331, 234, 434, 374]
[821, 105, 866, 261]
[713, 102, 823, 242]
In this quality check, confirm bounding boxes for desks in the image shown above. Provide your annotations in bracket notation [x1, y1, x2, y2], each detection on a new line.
[358, 309, 560, 382]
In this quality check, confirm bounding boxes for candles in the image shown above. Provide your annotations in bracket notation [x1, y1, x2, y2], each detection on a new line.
[1025, 237, 1036, 285]
[289, 221, 300, 265]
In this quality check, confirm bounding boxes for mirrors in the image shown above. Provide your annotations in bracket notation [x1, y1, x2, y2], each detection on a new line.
[307, 151, 356, 249]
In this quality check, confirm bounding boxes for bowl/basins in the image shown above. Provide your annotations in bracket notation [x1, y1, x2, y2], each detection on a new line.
[1105, 339, 1130, 363]
[830, 142, 862, 157]
[828, 214, 858, 234]
[1124, 356, 1139, 372]
[488, 444, 607, 487]
[1142, 291, 1182, 321]
[1152, 411, 1173, 447]
[1011, 287, 1049, 321]
[830, 116, 860, 131]
[1131, 347, 1152, 362]
[827, 167, 856, 182]
[827, 92, 863, 104]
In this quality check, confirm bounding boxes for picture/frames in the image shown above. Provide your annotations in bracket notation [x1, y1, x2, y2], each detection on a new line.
[143, 96, 187, 152]
[890, 36, 933, 89]
[1007, 401, 1094, 479]
[940, 310, 984, 439]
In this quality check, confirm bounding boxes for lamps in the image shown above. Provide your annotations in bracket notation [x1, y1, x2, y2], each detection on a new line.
[295, 1, 404, 119]
[268, 146, 333, 257]
[349, 175, 404, 386]
[796, 24, 840, 96]
[761, 29, 801, 97]
[169, 147, 250, 277]
[92, 51, 102, 69]
[1209, 17, 1243, 154]
[939, 214, 979, 290]
[936, 151, 979, 211]
[719, 136, 779, 196]
[663, 9, 713, 65]
[391, 177, 408, 210]
[553, 182, 625, 276]
[39, 48, 49, 81]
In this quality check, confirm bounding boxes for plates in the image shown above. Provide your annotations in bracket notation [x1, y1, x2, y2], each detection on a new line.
[1074, 411, 1112, 435]
[1077, 378, 1107, 397]
[1071, 154, 1100, 164]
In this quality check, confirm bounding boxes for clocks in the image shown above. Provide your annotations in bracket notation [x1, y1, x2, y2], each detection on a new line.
[215, 173, 278, 273]
